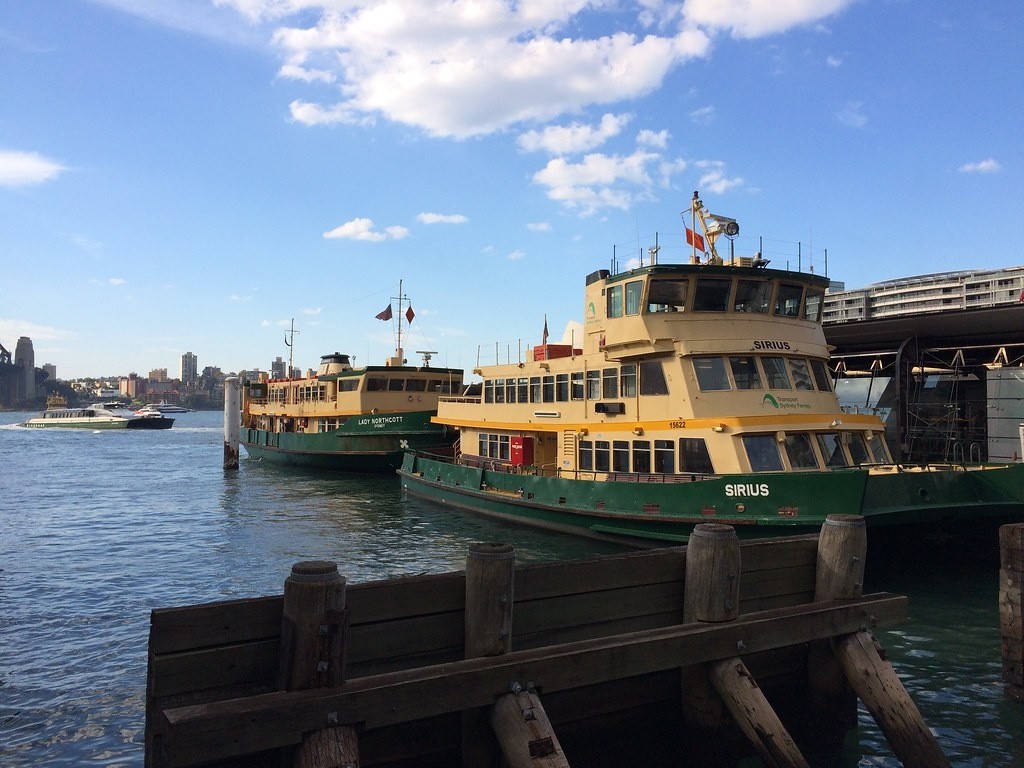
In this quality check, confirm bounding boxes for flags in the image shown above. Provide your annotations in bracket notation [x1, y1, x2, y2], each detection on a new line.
[686, 229, 704, 251]
[542, 321, 549, 345]
[405, 308, 414, 322]
[375, 304, 392, 321]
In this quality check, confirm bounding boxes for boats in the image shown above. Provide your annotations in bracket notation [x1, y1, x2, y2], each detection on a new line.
[46, 390, 68, 410]
[186, 409, 197, 412]
[395, 190, 1024, 551]
[238, 279, 466, 474]
[15, 400, 176, 430]
[145, 398, 186, 413]
[132, 408, 163, 418]
